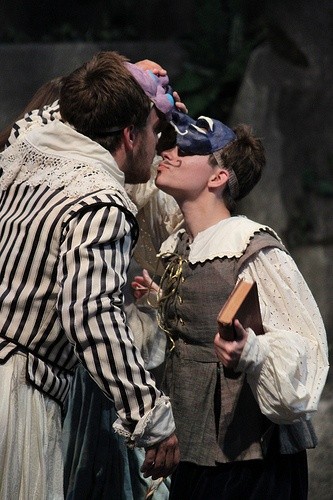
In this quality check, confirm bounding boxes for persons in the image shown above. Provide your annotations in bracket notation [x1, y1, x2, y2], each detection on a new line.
[0, 75, 189, 500]
[132, 115, 329, 500]
[0, 51, 180, 500]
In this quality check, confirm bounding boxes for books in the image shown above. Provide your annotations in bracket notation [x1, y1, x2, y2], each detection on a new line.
[217, 278, 265, 379]
